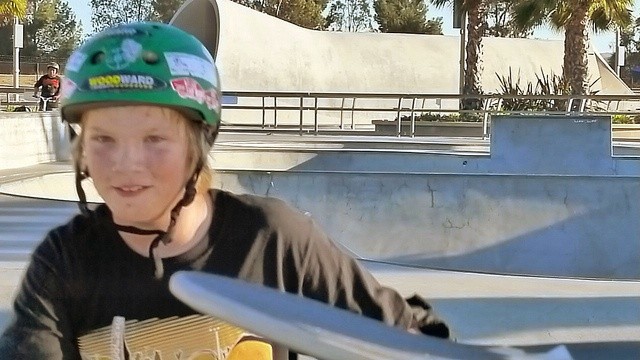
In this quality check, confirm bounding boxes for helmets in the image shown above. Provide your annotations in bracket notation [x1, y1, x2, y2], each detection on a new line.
[47, 62, 59, 72]
[62, 20, 221, 125]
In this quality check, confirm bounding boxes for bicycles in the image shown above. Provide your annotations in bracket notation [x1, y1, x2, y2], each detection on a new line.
[33, 95, 56, 111]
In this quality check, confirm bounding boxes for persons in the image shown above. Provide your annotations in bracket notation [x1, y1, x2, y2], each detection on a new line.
[1, 18, 457, 359]
[33, 62, 63, 111]
